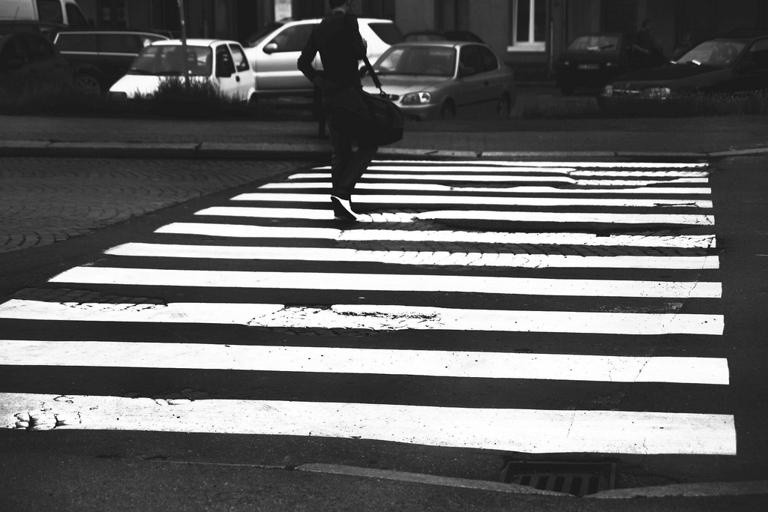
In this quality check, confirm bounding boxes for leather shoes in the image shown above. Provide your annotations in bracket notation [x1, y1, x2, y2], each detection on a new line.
[329, 193, 358, 220]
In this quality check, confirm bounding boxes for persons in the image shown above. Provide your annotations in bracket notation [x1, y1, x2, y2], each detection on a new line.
[632, 16, 654, 69]
[295, 1, 383, 220]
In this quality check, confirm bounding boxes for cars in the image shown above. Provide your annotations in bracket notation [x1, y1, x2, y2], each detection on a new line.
[554, 27, 666, 90]
[596, 27, 767, 111]
[107, 38, 258, 105]
[359, 40, 514, 123]
[398, 30, 489, 45]
[46, 24, 177, 87]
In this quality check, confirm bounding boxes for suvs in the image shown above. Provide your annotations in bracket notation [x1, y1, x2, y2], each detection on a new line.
[195, 15, 407, 105]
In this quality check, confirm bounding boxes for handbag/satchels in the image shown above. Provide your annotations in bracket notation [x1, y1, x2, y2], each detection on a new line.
[329, 61, 406, 148]
